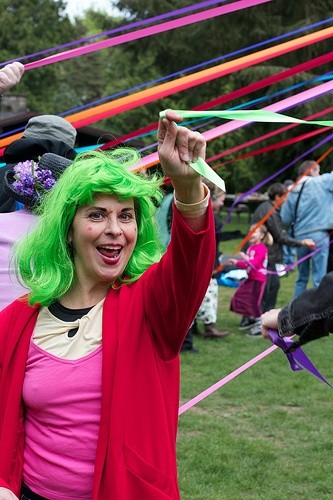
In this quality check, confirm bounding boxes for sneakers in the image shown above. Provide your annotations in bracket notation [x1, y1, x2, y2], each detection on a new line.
[238, 318, 261, 330]
[246, 328, 262, 337]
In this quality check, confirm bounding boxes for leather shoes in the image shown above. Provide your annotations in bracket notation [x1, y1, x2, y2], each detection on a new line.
[204, 326, 228, 338]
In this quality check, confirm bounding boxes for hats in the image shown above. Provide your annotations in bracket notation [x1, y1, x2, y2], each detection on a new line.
[23, 115, 77, 149]
[4, 153, 73, 206]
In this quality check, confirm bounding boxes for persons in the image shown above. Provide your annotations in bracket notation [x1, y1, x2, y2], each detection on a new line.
[0, 109, 216, 500]
[262, 271, 333, 338]
[157, 186, 200, 353]
[0, 61, 78, 313]
[230, 224, 268, 337]
[280, 160, 333, 302]
[240, 183, 315, 314]
[186, 278, 229, 338]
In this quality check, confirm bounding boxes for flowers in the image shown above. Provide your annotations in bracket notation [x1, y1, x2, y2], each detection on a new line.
[11, 156, 55, 198]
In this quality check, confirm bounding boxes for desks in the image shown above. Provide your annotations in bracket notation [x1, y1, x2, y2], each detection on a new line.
[217, 193, 269, 225]
